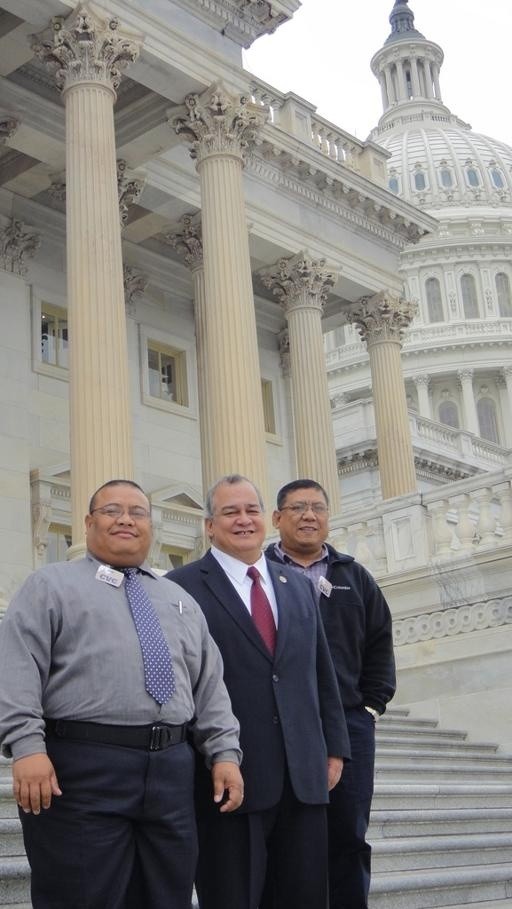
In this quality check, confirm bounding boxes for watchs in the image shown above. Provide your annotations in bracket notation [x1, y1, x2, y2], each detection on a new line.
[364, 706, 380, 721]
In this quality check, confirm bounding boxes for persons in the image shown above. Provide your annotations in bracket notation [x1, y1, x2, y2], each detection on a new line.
[160, 476, 353, 909]
[264, 480, 397, 909]
[0, 481, 244, 909]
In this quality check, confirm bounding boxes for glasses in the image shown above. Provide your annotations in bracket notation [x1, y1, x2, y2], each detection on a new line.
[278, 502, 327, 514]
[89, 502, 149, 519]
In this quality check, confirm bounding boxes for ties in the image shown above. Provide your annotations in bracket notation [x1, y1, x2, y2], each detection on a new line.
[116, 567, 176, 707]
[245, 566, 278, 657]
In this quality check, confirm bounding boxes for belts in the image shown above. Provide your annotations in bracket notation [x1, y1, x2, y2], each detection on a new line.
[42, 717, 192, 752]
